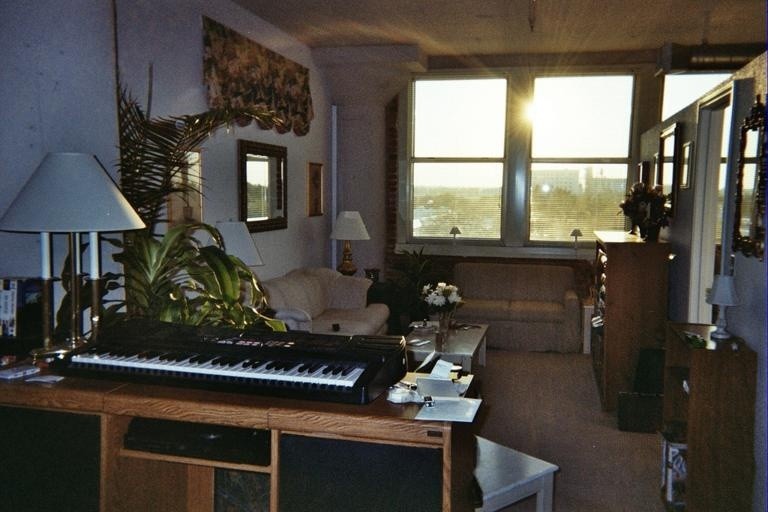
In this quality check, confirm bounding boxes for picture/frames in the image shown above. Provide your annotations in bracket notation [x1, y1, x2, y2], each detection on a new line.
[636, 121, 694, 219]
[304, 160, 325, 218]
[165, 150, 205, 233]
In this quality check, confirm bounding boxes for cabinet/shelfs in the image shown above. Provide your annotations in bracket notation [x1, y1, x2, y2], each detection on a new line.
[658, 322, 760, 512]
[593, 229, 672, 412]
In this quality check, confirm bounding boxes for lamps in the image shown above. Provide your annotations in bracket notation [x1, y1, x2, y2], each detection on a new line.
[570, 228, 583, 250]
[0, 151, 150, 360]
[705, 274, 740, 340]
[328, 210, 371, 276]
[449, 227, 462, 245]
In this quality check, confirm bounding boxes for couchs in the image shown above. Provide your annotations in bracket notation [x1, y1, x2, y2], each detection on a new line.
[453, 261, 584, 355]
[256, 266, 390, 335]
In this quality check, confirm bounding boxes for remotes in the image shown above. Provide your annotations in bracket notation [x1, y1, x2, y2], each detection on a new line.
[686, 332, 706, 348]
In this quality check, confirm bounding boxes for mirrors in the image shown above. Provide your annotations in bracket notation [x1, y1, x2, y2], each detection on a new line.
[730, 94, 767, 264]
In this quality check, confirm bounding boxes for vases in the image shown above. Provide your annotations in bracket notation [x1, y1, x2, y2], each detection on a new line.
[629, 221, 637, 235]
[646, 226, 660, 242]
[437, 307, 451, 342]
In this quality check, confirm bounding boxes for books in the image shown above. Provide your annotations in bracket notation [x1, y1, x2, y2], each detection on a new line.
[1, 276, 10, 337]
[10, 275, 43, 338]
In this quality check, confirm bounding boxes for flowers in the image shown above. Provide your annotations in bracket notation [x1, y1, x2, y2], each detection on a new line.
[625, 180, 671, 242]
[417, 274, 467, 312]
[615, 191, 642, 228]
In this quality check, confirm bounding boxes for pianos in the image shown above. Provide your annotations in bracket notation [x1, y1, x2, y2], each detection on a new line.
[64, 317, 409, 406]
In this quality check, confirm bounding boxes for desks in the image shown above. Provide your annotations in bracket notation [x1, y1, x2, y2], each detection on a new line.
[404, 321, 490, 373]
[0, 357, 457, 512]
[474, 431, 561, 512]
[367, 279, 392, 310]
